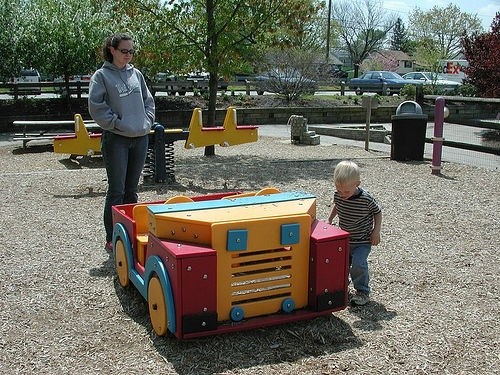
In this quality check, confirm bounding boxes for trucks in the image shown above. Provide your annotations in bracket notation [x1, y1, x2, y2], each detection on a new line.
[431, 59, 471, 82]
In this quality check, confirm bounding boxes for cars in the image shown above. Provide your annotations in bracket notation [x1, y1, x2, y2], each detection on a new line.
[217, 78, 228, 92]
[255, 67, 317, 97]
[349, 71, 425, 96]
[55, 71, 92, 94]
[402, 72, 464, 94]
[146, 63, 211, 96]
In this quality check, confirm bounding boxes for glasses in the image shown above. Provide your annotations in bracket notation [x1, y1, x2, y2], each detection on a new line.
[116, 47, 136, 54]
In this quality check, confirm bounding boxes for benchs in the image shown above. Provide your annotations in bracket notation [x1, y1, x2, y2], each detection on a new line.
[15, 133, 75, 137]
[13, 136, 55, 149]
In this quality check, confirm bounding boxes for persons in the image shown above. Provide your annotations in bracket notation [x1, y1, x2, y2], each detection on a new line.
[88, 34, 155, 254]
[326, 160, 382, 305]
[443, 66, 447, 73]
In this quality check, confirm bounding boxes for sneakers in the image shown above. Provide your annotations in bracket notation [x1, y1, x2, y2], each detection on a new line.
[106, 240, 114, 250]
[350, 291, 370, 305]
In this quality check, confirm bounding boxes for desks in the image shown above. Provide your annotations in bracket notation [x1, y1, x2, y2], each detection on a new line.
[13, 120, 99, 137]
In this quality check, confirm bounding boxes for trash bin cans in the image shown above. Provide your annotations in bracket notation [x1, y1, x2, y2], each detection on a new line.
[391, 100, 428, 160]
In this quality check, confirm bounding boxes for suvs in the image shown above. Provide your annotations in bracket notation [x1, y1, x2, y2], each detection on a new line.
[9, 66, 41, 96]
